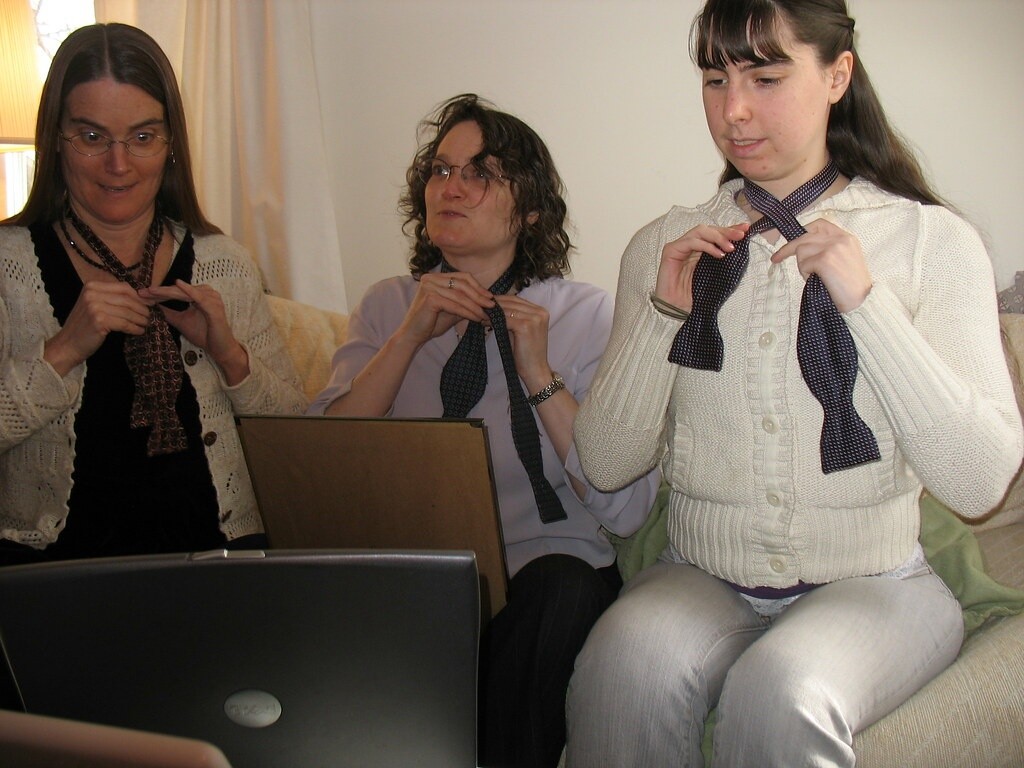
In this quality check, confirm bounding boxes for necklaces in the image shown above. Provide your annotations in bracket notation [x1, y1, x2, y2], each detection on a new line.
[453, 325, 493, 340]
[56, 206, 165, 273]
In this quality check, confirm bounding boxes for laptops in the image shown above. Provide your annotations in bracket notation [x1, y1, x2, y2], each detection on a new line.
[0, 549, 481, 768]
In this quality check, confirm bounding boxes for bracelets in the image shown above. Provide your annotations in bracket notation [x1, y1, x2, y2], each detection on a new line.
[649, 295, 690, 320]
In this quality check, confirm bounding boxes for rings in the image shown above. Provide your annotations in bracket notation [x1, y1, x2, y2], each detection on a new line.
[448, 276, 457, 289]
[509, 309, 517, 319]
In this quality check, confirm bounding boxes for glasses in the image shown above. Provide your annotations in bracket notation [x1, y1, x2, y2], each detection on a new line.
[55, 126, 173, 157]
[414, 158, 518, 193]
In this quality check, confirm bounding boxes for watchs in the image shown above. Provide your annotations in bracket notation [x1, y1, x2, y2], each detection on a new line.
[527, 372, 565, 407]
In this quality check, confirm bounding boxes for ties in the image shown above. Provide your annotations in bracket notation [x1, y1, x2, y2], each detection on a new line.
[63, 188, 188, 456]
[668, 157, 882, 474]
[441, 258, 567, 524]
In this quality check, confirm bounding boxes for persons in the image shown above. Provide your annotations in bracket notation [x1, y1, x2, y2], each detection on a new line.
[1, 24, 304, 562]
[565, 0, 1024, 768]
[306, 107, 667, 768]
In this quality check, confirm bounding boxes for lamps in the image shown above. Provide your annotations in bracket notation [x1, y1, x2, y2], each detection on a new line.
[0, 0, 48, 220]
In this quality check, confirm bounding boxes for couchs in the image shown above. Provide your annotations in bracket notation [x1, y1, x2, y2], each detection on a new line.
[557, 309, 1023, 767]
